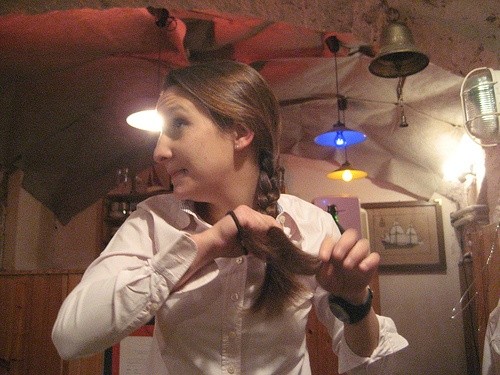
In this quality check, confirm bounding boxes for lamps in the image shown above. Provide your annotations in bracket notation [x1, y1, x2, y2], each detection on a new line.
[125, 30, 167, 133]
[313, 33, 368, 182]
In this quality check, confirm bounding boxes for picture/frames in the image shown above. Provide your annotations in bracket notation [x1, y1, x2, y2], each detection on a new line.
[360, 201, 446, 270]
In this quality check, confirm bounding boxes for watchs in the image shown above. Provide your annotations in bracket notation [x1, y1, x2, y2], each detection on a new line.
[327, 286, 373, 326]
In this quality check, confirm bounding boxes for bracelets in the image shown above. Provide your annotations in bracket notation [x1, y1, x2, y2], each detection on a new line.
[228, 210, 242, 234]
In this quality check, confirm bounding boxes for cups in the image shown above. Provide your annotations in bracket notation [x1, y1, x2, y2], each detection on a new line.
[111, 168, 132, 194]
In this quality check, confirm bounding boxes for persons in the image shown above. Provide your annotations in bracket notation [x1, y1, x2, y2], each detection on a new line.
[51, 59, 409, 375]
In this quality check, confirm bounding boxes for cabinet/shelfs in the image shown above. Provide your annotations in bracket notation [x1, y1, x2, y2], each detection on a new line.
[104, 192, 148, 250]
[0, 270, 104, 375]
